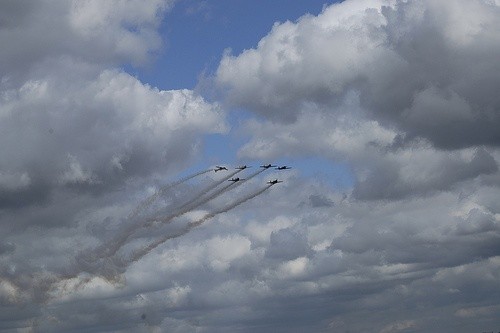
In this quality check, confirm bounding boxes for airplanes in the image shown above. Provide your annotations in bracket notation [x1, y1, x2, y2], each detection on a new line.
[228, 177, 246, 182]
[259, 163, 277, 169]
[267, 179, 283, 185]
[274, 166, 292, 170]
[214, 166, 228, 173]
[235, 164, 253, 170]
